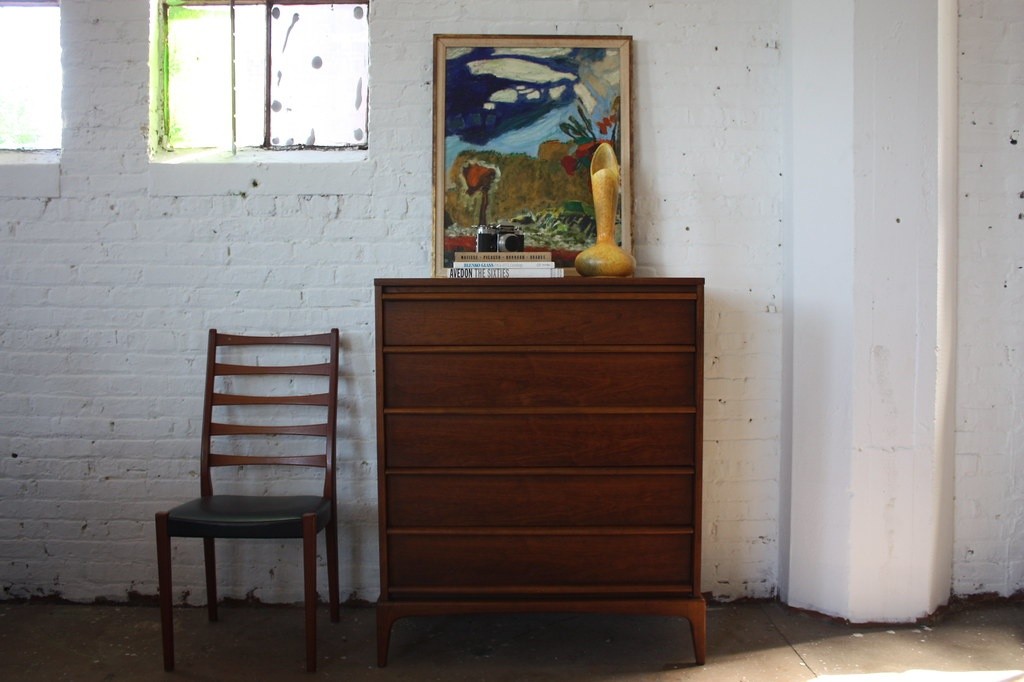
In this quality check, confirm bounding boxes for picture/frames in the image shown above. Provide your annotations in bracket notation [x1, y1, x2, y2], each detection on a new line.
[431, 33, 632, 280]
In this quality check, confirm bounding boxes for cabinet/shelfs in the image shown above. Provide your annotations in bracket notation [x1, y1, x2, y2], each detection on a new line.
[373, 277, 707, 668]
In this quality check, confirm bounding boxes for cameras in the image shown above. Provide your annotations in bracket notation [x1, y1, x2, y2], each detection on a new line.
[476, 223, 524, 253]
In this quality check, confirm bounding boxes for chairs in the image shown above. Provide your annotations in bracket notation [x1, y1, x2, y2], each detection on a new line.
[155, 327, 340, 673]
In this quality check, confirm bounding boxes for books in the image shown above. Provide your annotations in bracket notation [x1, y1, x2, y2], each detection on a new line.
[447, 252, 564, 278]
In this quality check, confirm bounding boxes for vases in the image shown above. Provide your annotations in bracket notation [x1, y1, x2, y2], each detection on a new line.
[574, 142, 637, 280]
